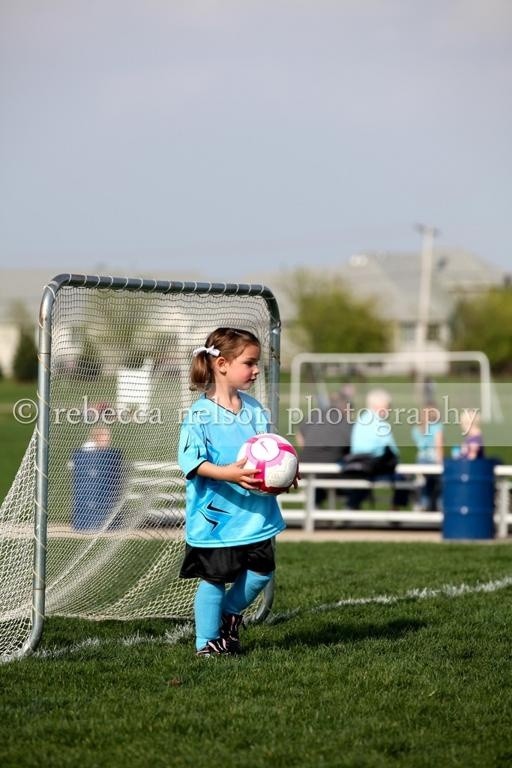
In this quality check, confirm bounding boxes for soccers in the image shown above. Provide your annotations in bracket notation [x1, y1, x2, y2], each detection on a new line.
[237, 432, 299, 495]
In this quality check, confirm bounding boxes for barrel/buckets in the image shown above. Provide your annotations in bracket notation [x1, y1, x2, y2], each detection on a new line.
[440, 457, 499, 540]
[73, 450, 123, 528]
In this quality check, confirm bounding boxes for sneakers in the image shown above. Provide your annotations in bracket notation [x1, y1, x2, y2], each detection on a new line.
[197, 642, 223, 659]
[224, 609, 243, 652]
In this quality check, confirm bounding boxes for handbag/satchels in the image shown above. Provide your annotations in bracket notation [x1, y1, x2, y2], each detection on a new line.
[343, 447, 398, 480]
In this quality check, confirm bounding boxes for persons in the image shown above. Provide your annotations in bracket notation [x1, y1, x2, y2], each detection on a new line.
[406, 399, 445, 512]
[349, 388, 412, 528]
[176, 327, 302, 661]
[294, 392, 354, 511]
[456, 408, 486, 461]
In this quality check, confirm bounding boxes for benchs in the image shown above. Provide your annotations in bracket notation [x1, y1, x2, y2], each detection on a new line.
[126, 459, 512, 540]
